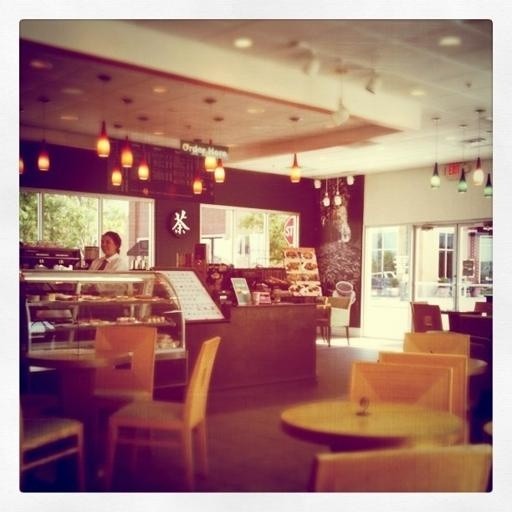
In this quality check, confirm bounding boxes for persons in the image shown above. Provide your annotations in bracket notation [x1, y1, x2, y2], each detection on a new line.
[72, 230, 130, 341]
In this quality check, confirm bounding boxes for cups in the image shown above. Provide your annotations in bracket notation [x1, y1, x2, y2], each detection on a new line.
[336, 280, 353, 298]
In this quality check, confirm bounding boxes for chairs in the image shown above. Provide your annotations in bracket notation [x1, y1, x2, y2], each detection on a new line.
[327, 290, 355, 346]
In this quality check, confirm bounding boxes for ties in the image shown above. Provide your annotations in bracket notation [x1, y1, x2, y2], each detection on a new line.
[99, 260, 107, 270]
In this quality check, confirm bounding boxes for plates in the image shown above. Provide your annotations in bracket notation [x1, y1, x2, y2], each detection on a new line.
[332, 290, 356, 305]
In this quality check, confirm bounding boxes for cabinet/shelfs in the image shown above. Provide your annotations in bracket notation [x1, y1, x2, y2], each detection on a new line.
[19, 270, 188, 402]
[20, 245, 82, 270]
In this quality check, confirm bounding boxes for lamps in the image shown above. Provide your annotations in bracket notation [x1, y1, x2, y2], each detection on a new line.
[20, 116, 355, 207]
[292, 41, 389, 95]
[430, 110, 493, 197]
